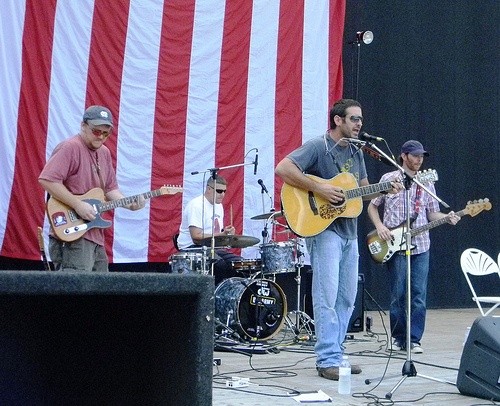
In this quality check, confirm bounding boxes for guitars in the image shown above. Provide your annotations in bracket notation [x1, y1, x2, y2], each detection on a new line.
[366, 198, 493, 264]
[46, 184, 185, 243]
[280, 168, 439, 238]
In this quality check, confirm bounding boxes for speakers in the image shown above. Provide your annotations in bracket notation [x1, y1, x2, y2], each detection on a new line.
[0, 269, 214, 406]
[456, 316, 500, 402]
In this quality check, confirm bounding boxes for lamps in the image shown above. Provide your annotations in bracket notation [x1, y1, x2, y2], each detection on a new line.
[346, 30, 373, 100]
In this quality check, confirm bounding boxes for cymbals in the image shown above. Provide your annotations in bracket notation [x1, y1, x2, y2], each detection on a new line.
[200, 234, 260, 249]
[250, 210, 284, 220]
[275, 229, 291, 235]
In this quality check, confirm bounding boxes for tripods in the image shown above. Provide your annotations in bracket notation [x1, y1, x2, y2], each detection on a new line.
[366, 140, 456, 400]
[283, 239, 314, 341]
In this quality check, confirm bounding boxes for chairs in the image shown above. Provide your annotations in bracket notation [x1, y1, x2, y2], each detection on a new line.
[37, 225, 52, 270]
[460, 248, 500, 316]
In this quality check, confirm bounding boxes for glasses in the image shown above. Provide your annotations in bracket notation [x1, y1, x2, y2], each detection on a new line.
[350, 116, 363, 123]
[207, 184, 226, 193]
[85, 122, 109, 137]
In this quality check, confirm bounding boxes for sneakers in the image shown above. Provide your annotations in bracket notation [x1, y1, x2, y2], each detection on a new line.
[317, 367, 339, 381]
[351, 365, 362, 374]
[390, 340, 404, 351]
[410, 343, 423, 353]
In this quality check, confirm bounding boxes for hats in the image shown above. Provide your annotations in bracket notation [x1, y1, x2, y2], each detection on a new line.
[83, 106, 114, 128]
[401, 140, 427, 156]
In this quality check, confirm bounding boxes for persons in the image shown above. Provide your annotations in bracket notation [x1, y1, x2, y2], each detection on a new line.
[367, 140, 461, 354]
[38, 105, 145, 273]
[274, 99, 403, 380]
[176, 174, 239, 285]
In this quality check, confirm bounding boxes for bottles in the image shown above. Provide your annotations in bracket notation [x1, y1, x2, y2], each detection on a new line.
[337, 356, 351, 395]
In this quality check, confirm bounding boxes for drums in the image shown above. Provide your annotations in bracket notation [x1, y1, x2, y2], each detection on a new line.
[213, 276, 288, 341]
[168, 252, 210, 276]
[230, 258, 262, 271]
[258, 239, 296, 275]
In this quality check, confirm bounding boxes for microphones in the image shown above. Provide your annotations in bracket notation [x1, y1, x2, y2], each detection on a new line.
[254, 154, 258, 175]
[359, 131, 383, 142]
[258, 179, 271, 198]
